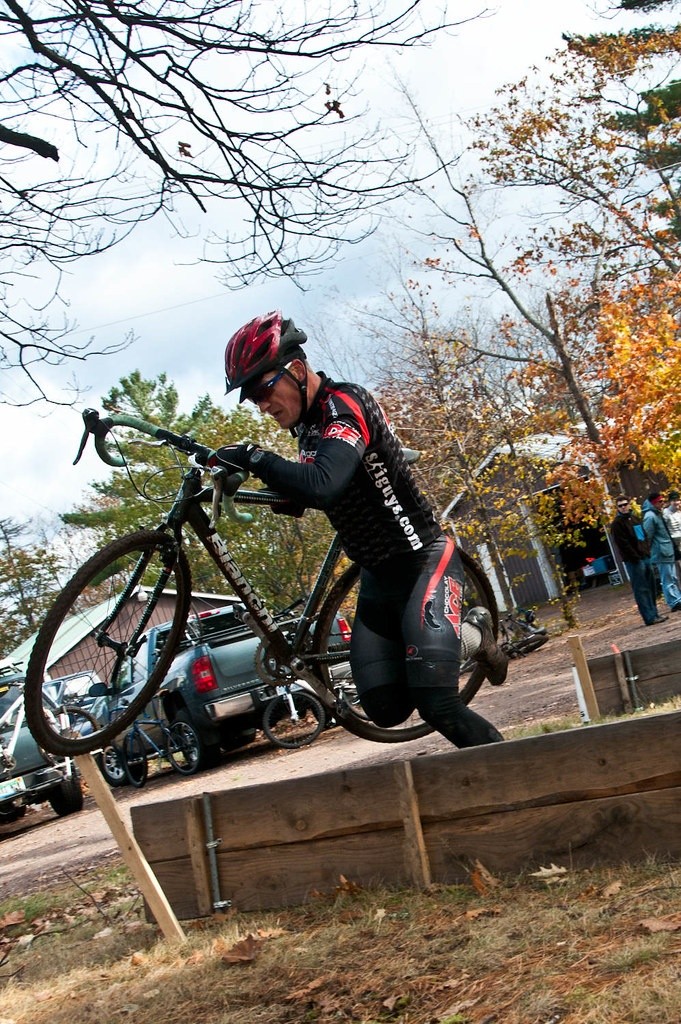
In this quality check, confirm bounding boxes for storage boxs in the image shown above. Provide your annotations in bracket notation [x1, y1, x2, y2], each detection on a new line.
[583, 565, 595, 577]
[590, 553, 615, 574]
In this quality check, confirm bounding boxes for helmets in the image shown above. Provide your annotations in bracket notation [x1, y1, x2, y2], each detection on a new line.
[223, 308, 307, 395]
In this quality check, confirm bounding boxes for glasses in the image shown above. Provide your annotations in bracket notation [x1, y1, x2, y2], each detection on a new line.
[657, 499, 664, 503]
[248, 369, 287, 405]
[617, 503, 629, 508]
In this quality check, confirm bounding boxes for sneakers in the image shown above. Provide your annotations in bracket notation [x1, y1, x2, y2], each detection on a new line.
[462, 606, 509, 685]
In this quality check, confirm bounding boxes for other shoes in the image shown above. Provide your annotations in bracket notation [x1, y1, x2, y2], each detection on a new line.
[647, 616, 664, 625]
[657, 613, 669, 620]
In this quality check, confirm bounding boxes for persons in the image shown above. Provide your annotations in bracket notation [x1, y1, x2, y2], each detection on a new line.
[610, 496, 668, 627]
[641, 493, 680, 612]
[662, 492, 681, 593]
[204, 307, 508, 751]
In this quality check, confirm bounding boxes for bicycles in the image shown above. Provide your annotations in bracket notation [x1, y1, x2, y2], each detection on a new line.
[456, 607, 549, 677]
[23, 403, 504, 755]
[260, 661, 372, 748]
[113, 687, 202, 789]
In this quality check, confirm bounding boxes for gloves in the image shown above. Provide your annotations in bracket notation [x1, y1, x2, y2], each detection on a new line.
[208, 442, 273, 478]
[259, 486, 306, 518]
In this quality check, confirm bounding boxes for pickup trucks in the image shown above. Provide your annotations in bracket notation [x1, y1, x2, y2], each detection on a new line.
[88, 600, 352, 786]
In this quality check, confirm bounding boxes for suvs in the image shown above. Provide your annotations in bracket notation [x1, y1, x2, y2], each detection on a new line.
[48, 671, 103, 727]
[1, 663, 85, 822]
